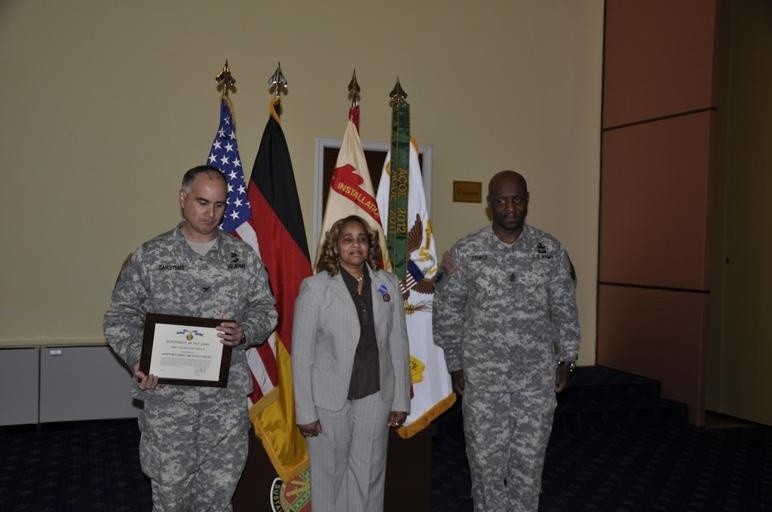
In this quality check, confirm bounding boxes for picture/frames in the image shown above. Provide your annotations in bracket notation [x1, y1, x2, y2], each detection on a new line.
[138, 312, 236, 389]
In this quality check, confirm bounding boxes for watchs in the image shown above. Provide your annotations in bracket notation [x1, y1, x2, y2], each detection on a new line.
[558, 359, 576, 373]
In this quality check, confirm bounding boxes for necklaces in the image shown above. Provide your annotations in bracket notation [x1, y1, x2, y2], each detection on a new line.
[356, 274, 366, 283]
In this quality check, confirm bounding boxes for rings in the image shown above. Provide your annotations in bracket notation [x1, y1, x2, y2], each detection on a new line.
[394, 422, 400, 427]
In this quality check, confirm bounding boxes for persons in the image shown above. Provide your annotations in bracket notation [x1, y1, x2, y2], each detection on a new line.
[432, 169, 580, 512]
[290, 215, 412, 512]
[100, 165, 279, 512]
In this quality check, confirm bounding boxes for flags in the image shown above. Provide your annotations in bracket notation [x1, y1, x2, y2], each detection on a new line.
[245, 97, 310, 481]
[376, 136, 457, 438]
[312, 106, 393, 273]
[206, 95, 278, 410]
